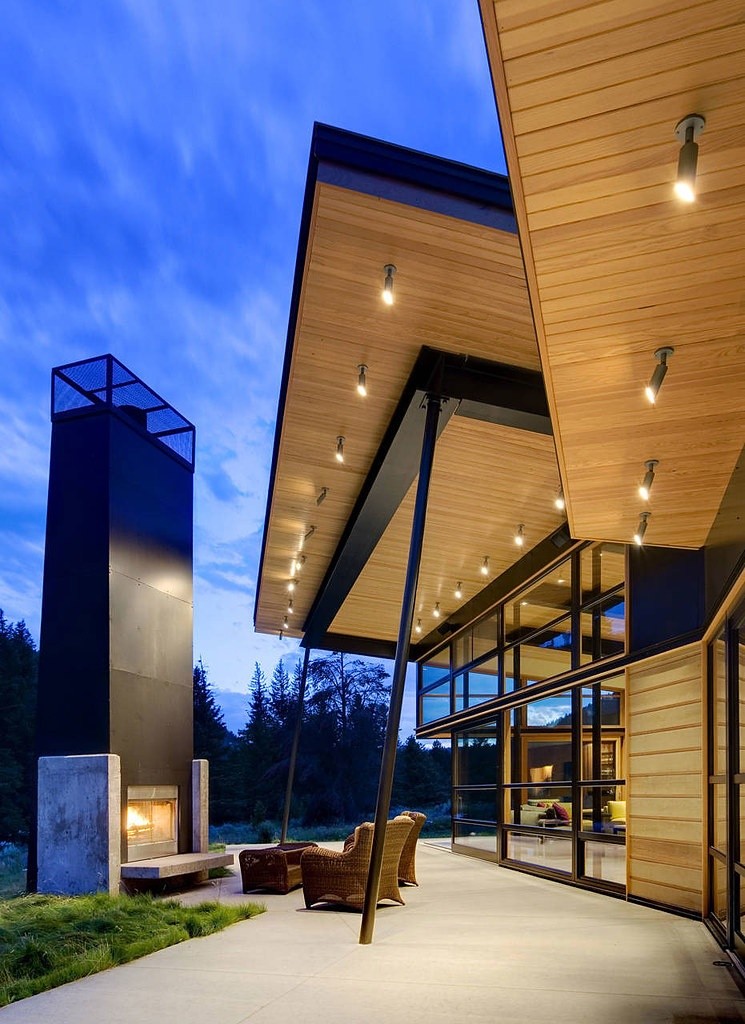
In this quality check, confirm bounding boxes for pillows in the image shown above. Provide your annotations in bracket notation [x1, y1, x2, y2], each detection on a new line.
[553, 803, 569, 821]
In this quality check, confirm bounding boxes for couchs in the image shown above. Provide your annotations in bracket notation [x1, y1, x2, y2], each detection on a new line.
[300, 811, 427, 913]
[512, 801, 626, 836]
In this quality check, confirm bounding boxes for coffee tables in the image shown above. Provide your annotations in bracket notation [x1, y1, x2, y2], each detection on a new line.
[538, 819, 571, 844]
[239, 843, 318, 895]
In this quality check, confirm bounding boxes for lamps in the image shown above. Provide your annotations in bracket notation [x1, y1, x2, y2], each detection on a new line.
[284, 616, 289, 628]
[317, 487, 329, 506]
[357, 365, 368, 396]
[336, 436, 345, 462]
[674, 113, 705, 199]
[305, 526, 317, 540]
[279, 630, 284, 640]
[296, 556, 307, 570]
[634, 512, 651, 545]
[382, 264, 396, 303]
[434, 602, 440, 617]
[640, 460, 658, 498]
[482, 556, 490, 575]
[456, 582, 463, 598]
[515, 524, 525, 545]
[288, 599, 294, 613]
[645, 346, 674, 402]
[416, 619, 422, 632]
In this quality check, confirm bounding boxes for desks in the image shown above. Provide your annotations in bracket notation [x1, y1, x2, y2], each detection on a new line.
[121, 853, 234, 878]
